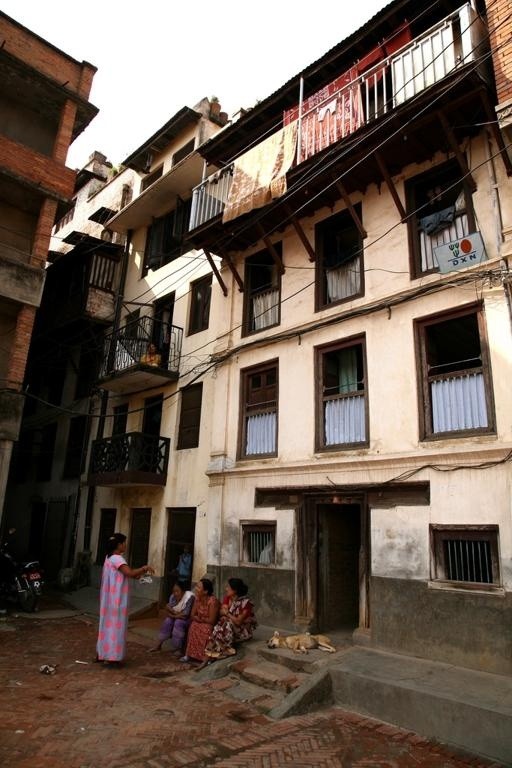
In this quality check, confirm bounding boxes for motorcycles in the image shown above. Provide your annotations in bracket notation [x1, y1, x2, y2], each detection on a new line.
[1, 526, 50, 614]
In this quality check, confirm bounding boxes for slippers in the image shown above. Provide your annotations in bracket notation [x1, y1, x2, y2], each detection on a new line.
[146, 647, 192, 663]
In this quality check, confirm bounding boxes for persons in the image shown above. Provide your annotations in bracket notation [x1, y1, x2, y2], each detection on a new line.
[147, 579, 195, 660]
[141, 342, 162, 367]
[174, 543, 192, 591]
[93, 533, 154, 666]
[178, 578, 222, 663]
[193, 578, 258, 672]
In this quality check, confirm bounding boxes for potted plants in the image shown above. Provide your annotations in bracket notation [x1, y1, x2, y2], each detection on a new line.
[210, 96, 221, 117]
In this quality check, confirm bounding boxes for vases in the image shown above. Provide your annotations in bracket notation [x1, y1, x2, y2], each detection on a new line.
[218, 111, 228, 125]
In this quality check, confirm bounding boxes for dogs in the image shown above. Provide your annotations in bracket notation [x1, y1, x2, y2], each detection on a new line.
[264, 629, 337, 655]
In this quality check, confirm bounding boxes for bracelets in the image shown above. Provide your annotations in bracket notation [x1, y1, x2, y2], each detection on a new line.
[142, 565, 148, 572]
